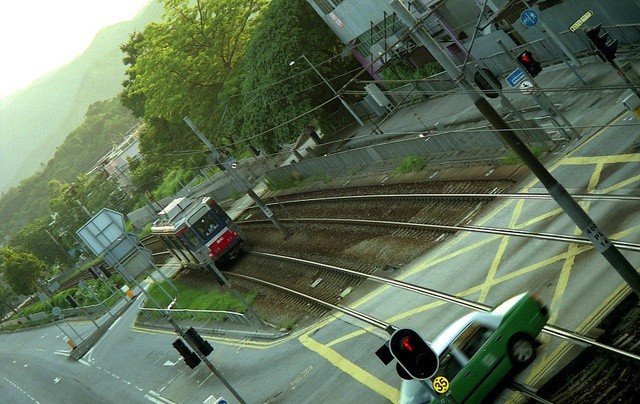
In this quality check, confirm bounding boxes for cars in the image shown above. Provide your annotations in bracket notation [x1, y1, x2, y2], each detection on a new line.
[401, 293, 548, 404]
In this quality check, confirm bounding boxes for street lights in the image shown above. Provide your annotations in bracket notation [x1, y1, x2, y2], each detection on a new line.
[288, 55, 363, 126]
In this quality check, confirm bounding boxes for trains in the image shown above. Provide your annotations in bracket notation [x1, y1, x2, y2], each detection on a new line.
[151, 197, 245, 269]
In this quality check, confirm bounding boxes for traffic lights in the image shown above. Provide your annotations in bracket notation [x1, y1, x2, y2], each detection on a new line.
[171, 328, 212, 369]
[475, 70, 502, 98]
[87, 268, 100, 280]
[518, 51, 543, 77]
[65, 295, 78, 306]
[100, 264, 112, 278]
[375, 330, 439, 381]
[588, 24, 619, 60]
